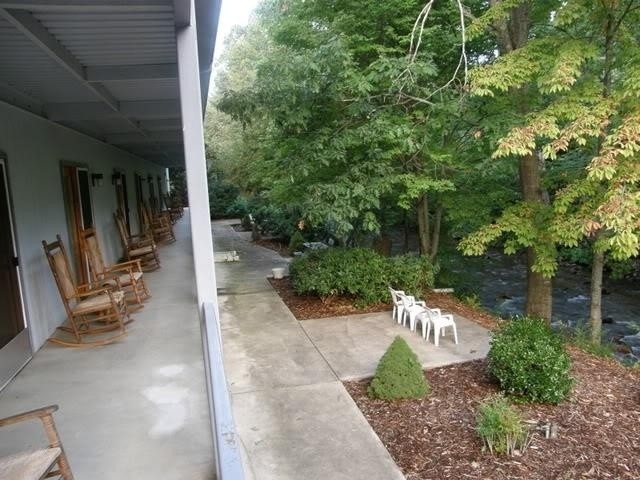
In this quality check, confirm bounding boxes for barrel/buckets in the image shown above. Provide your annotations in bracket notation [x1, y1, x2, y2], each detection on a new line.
[272, 267, 286, 280]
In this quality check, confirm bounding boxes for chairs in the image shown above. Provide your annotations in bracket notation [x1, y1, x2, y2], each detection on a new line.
[387, 287, 459, 347]
[112, 208, 163, 272]
[0, 405, 74, 479]
[136, 192, 183, 246]
[42, 233, 135, 349]
[77, 228, 152, 312]
